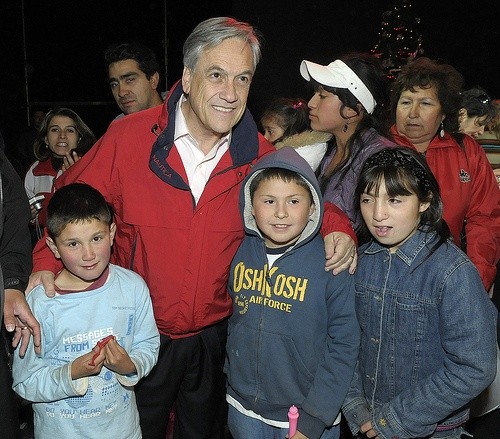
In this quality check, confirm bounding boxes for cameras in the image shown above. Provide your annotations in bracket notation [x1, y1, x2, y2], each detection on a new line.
[28, 195, 45, 210]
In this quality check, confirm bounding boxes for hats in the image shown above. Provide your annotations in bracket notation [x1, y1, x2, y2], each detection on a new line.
[300, 58, 377, 115]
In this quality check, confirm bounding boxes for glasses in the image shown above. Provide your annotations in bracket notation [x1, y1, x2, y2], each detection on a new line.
[472, 93, 491, 106]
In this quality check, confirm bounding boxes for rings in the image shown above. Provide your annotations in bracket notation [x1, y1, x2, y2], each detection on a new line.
[21, 327, 27, 330]
[351, 255, 355, 259]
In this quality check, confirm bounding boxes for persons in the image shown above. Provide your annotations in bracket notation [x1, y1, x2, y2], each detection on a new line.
[0, 42, 500, 439]
[25, 18, 358, 439]
[223, 146, 360, 439]
